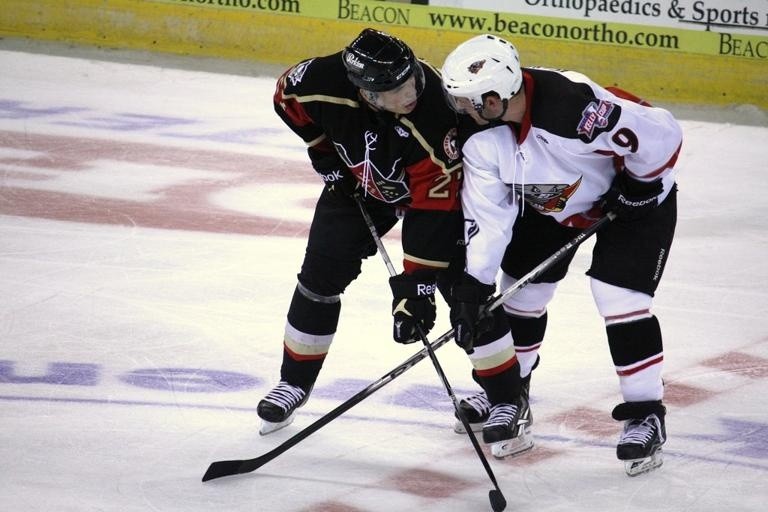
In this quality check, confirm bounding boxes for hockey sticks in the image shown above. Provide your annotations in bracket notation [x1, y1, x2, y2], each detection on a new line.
[202, 212, 615, 484]
[353, 192, 507, 512]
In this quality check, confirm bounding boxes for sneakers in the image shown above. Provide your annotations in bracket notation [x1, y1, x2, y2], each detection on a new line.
[257, 381, 307, 422]
[617, 413, 666, 459]
[455, 392, 533, 443]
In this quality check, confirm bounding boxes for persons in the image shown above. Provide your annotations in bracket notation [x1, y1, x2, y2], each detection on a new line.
[440, 31, 683, 462]
[256, 27, 537, 445]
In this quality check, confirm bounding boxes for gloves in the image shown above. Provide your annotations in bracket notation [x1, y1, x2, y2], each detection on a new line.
[600, 170, 664, 222]
[308, 138, 354, 197]
[389, 270, 435, 343]
[449, 286, 478, 351]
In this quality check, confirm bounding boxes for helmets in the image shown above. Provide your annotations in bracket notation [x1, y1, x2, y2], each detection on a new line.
[441, 34, 523, 109]
[342, 28, 414, 92]
